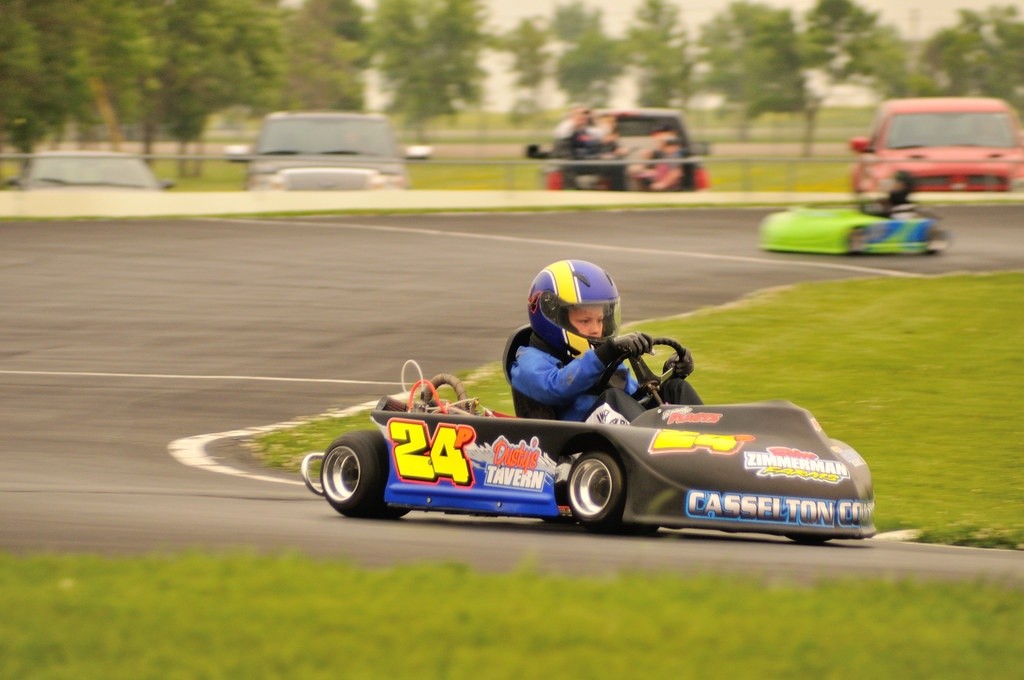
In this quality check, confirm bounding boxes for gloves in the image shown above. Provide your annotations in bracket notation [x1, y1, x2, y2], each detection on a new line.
[613, 331, 653, 355]
[664, 349, 694, 379]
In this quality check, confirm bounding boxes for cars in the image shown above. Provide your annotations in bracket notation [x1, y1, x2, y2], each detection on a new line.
[5, 148, 177, 194]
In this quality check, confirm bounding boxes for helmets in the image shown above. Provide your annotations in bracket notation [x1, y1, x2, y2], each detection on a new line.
[528, 259, 620, 358]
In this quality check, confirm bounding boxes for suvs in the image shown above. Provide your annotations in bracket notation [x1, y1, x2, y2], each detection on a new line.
[527, 103, 719, 195]
[227, 109, 434, 196]
[850, 97, 1024, 196]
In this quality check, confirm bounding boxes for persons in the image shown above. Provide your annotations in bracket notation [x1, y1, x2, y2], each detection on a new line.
[514, 260, 694, 426]
[628, 123, 684, 189]
[553, 102, 627, 190]
[872, 171, 914, 216]
